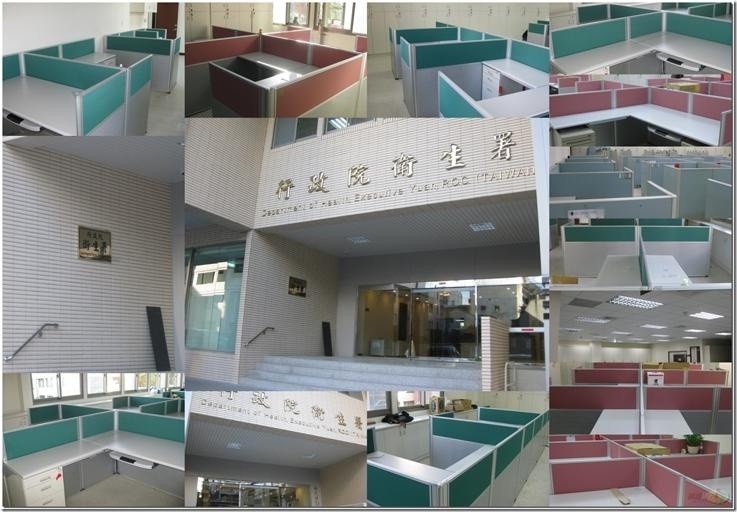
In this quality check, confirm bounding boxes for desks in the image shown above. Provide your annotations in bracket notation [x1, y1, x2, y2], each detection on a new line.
[367, 444, 497, 507]
[3, 406, 184, 507]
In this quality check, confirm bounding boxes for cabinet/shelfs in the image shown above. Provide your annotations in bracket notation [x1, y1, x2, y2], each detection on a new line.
[376, 410, 479, 460]
